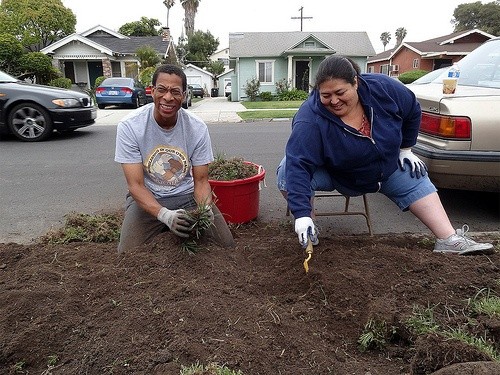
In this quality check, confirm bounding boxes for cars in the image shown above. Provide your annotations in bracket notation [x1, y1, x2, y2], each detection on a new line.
[146, 81, 205, 110]
[225, 82, 232, 97]
[410, 64, 500, 86]
[405, 37, 500, 194]
[0, 69, 98, 142]
[95, 77, 148, 109]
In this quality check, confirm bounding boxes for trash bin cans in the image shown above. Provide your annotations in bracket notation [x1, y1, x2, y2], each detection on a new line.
[211, 88, 219, 96]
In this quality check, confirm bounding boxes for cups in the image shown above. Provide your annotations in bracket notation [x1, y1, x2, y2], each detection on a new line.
[443, 78, 458, 94]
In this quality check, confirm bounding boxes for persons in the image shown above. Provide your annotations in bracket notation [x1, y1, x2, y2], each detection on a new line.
[276, 55, 495, 256]
[114, 65, 234, 254]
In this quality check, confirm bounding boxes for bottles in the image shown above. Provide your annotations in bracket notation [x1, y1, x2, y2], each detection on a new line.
[448, 62, 461, 78]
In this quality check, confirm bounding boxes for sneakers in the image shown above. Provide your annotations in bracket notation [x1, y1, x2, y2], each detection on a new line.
[312, 239, 319, 245]
[433, 224, 494, 255]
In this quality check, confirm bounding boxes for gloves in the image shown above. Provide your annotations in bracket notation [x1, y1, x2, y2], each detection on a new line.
[398, 148, 428, 180]
[205, 204, 215, 225]
[294, 217, 317, 246]
[158, 207, 195, 238]
